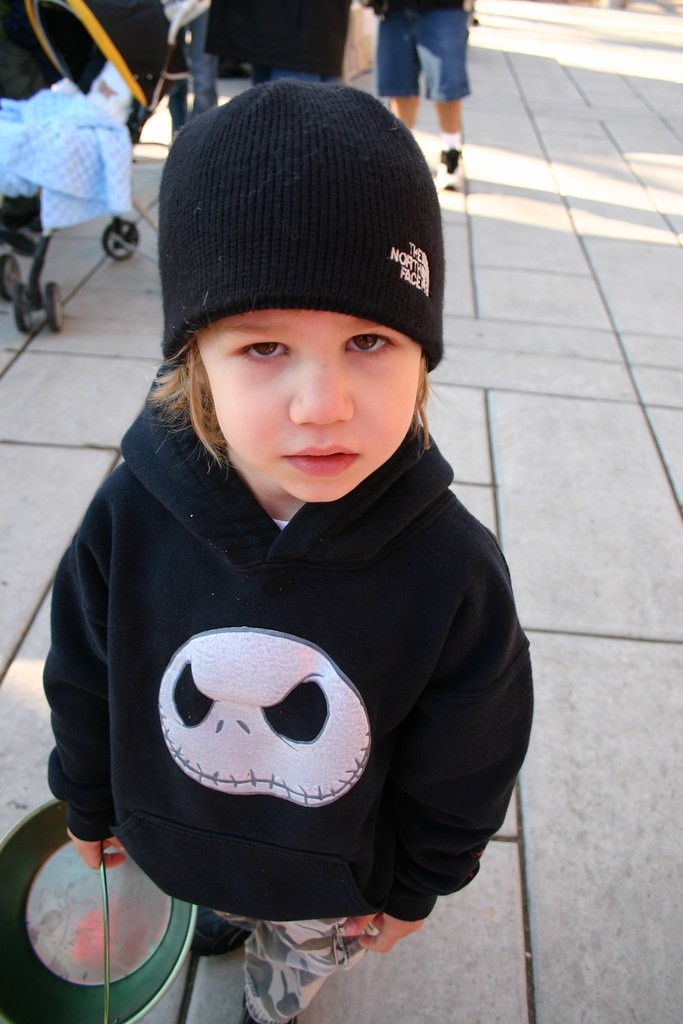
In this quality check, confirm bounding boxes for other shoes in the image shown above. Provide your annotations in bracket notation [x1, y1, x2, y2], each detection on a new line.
[190, 904, 252, 955]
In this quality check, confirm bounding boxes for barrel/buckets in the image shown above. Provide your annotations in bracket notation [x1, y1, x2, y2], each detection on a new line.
[0, 796, 201, 1024]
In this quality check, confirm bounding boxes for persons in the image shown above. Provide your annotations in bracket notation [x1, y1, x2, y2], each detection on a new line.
[1, 1, 480, 195]
[43, 78, 534, 1024]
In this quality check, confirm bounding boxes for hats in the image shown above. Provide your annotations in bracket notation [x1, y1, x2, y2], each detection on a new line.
[157, 77, 446, 371]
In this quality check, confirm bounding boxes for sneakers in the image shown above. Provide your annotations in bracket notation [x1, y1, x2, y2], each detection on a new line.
[437, 149, 464, 190]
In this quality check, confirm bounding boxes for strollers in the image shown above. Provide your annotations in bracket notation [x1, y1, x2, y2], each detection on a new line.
[0, 0, 211, 336]
